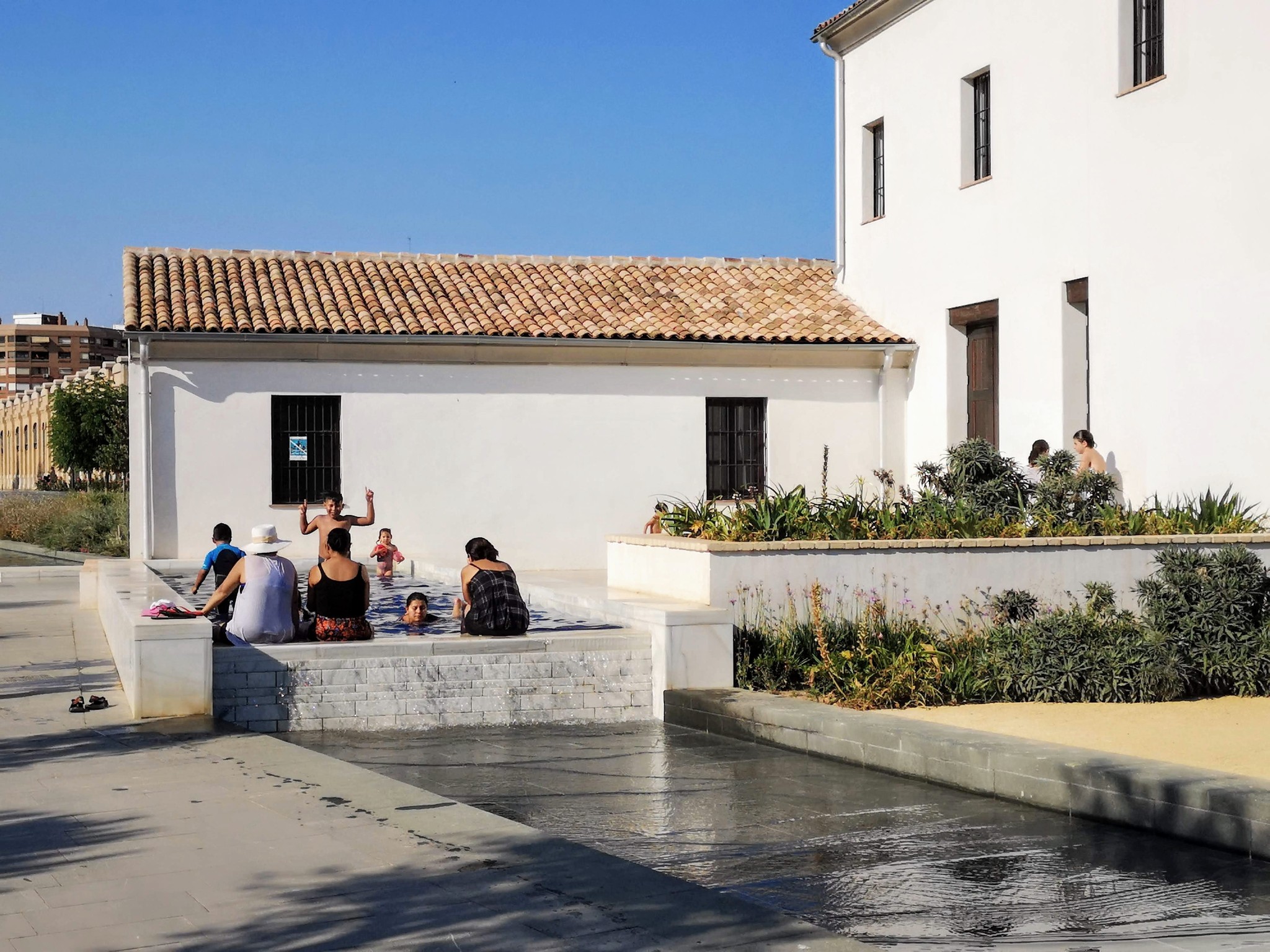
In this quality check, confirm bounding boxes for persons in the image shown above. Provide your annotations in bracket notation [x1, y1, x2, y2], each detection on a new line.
[643, 503, 668, 534]
[195, 525, 301, 646]
[370, 528, 398, 577]
[396, 592, 438, 622]
[452, 537, 530, 636]
[1020, 439, 1049, 528]
[1068, 430, 1106, 508]
[192, 523, 247, 620]
[306, 528, 374, 641]
[298, 485, 375, 563]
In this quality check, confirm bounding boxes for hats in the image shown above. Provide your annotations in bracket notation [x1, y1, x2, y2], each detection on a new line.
[242, 524, 292, 553]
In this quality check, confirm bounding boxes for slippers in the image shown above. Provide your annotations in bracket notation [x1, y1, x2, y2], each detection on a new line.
[141, 604, 167, 616]
[151, 610, 196, 619]
[166, 606, 204, 616]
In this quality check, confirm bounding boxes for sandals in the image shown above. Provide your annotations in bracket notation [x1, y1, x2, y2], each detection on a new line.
[85, 695, 108, 710]
[69, 697, 87, 712]
